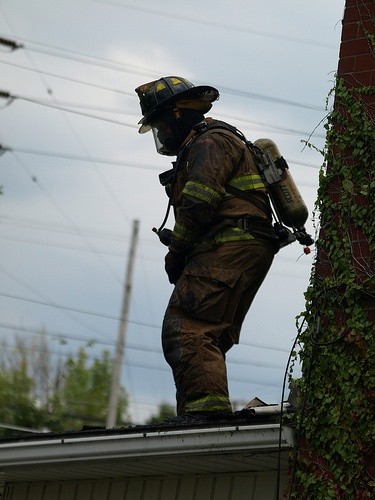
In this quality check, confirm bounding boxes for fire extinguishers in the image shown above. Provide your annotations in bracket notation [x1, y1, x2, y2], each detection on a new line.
[255, 138, 314, 255]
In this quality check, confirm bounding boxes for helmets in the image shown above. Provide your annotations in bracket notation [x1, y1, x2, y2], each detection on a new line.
[135, 74, 219, 134]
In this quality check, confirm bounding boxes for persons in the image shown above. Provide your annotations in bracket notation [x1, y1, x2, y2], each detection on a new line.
[135, 76, 281, 426]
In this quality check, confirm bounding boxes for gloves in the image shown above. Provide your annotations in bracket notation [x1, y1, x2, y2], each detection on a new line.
[163, 248, 183, 283]
